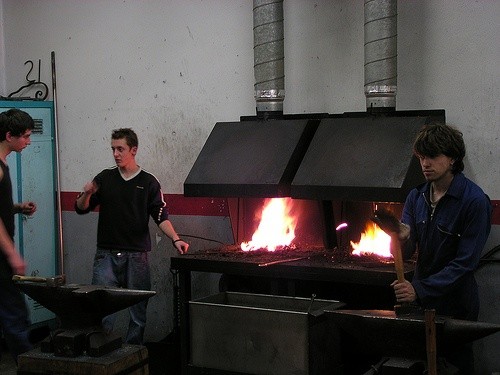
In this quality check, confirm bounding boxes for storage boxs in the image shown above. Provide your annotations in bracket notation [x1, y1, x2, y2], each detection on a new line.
[189, 291, 340, 375]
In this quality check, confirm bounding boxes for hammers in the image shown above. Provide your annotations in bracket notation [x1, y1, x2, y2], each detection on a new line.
[12, 275, 67, 288]
[391, 232, 411, 315]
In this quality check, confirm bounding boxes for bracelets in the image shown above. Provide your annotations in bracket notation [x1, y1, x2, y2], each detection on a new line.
[173, 239, 183, 243]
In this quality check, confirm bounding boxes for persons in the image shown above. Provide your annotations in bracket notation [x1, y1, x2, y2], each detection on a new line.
[389, 121, 493, 375]
[74, 128, 190, 346]
[0, 109, 38, 370]
